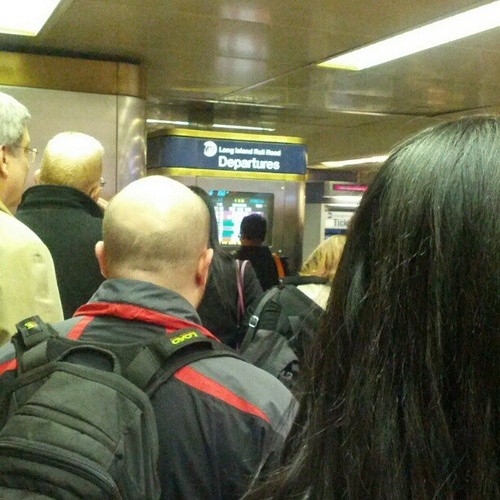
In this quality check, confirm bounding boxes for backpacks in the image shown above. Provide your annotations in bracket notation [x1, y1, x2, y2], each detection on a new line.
[0, 316, 250, 500]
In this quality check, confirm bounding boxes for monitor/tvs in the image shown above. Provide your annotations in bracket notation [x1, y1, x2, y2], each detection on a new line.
[211, 191, 274, 247]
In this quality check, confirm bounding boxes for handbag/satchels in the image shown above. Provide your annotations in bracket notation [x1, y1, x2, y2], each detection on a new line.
[237, 287, 300, 388]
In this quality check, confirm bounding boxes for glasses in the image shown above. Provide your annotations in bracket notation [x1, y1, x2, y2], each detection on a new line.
[238, 233, 242, 238]
[13, 144, 38, 164]
[99, 177, 106, 190]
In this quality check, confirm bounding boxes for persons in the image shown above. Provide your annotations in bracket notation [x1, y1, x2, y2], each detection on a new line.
[0, 92, 347, 361]
[240, 113, 500, 500]
[0, 174, 298, 500]
